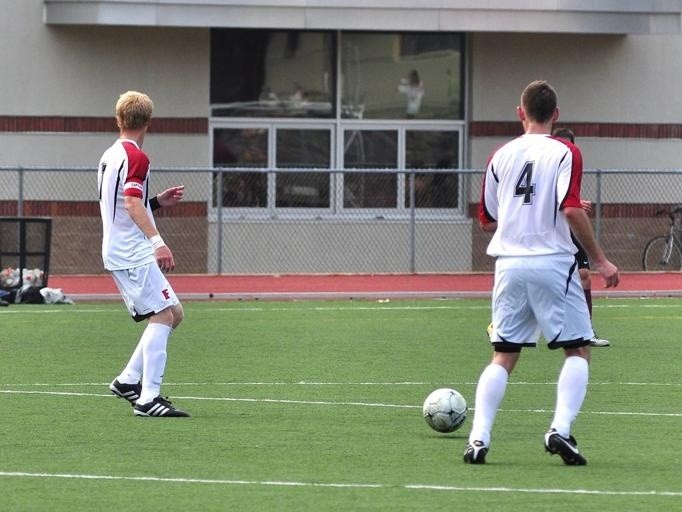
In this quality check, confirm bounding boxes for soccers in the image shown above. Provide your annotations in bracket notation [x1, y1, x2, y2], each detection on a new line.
[423, 388, 467, 433]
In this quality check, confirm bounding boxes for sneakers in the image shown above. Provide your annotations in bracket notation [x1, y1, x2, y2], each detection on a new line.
[545, 428, 587, 465]
[590, 336, 609, 346]
[464, 440, 488, 464]
[109, 376, 189, 417]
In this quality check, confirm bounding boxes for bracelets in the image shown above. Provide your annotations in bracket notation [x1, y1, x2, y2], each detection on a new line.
[149, 234, 166, 251]
[149, 196, 162, 212]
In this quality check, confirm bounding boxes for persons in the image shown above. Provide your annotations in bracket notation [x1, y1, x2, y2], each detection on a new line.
[98, 91, 189, 418]
[397, 71, 423, 120]
[553, 128, 611, 346]
[463, 80, 620, 466]
